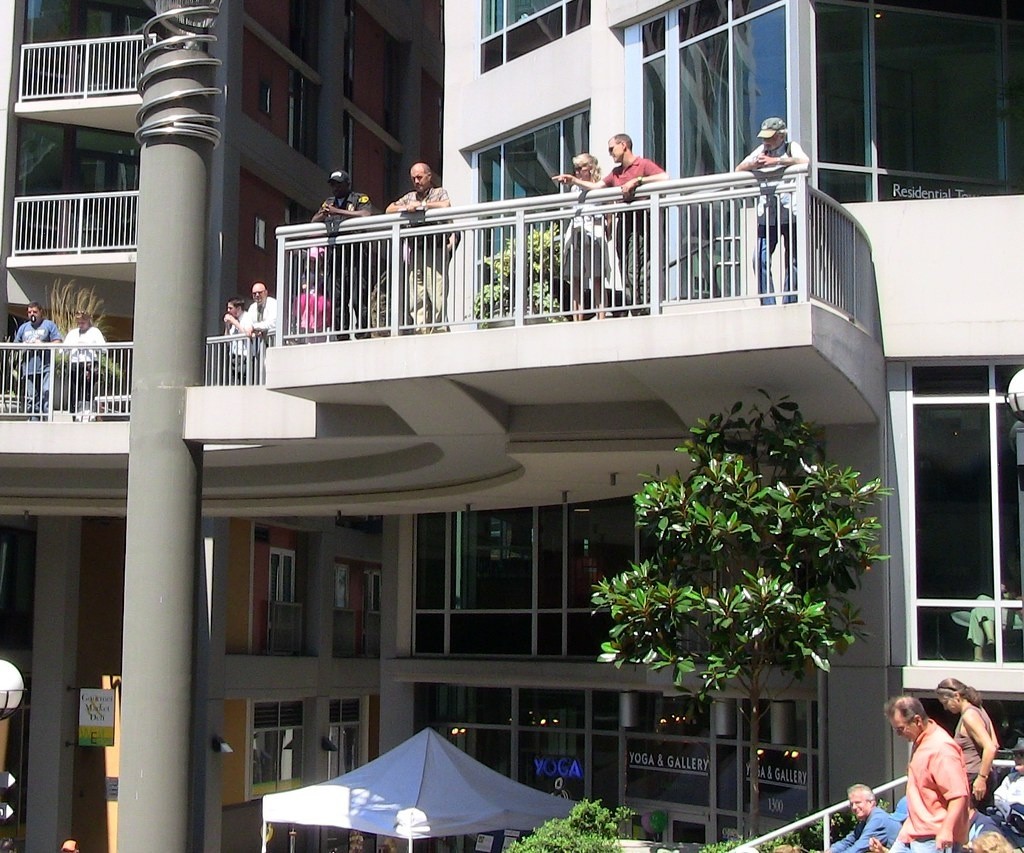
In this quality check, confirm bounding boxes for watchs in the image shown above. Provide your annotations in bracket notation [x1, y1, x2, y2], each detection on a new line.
[422, 200, 427, 211]
[637, 175, 642, 185]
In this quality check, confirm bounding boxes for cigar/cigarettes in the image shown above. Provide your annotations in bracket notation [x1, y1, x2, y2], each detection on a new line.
[33, 316, 35, 321]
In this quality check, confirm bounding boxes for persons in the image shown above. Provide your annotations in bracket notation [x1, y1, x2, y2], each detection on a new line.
[966, 548, 1023, 662]
[884, 695, 971, 853]
[311, 169, 372, 341]
[58, 310, 107, 422]
[243, 281, 277, 386]
[734, 117, 811, 306]
[994, 737, 1024, 846]
[967, 795, 1004, 842]
[552, 133, 669, 317]
[936, 678, 999, 814]
[223, 297, 256, 386]
[868, 831, 1013, 853]
[563, 153, 612, 322]
[60, 838, 80, 853]
[825, 783, 902, 853]
[12, 301, 63, 421]
[386, 162, 460, 334]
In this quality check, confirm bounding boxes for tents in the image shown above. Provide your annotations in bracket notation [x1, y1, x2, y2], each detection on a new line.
[262, 727, 582, 853]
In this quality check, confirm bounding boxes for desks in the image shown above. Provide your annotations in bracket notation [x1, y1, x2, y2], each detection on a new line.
[923, 607, 963, 661]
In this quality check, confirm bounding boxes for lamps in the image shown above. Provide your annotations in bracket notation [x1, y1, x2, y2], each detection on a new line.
[213, 736, 234, 753]
[771, 701, 797, 746]
[323, 736, 338, 752]
[714, 701, 737, 735]
[620, 692, 639, 727]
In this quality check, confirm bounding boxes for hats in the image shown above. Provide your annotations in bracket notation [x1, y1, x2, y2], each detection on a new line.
[757, 117, 786, 138]
[327, 169, 350, 183]
[1012, 737, 1024, 751]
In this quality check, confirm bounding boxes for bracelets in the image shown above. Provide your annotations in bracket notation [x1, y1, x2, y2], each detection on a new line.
[978, 773, 990, 779]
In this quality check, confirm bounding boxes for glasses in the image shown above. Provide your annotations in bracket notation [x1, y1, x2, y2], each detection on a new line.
[609, 143, 621, 152]
[575, 164, 586, 171]
[1013, 752, 1024, 758]
[937, 684, 956, 691]
